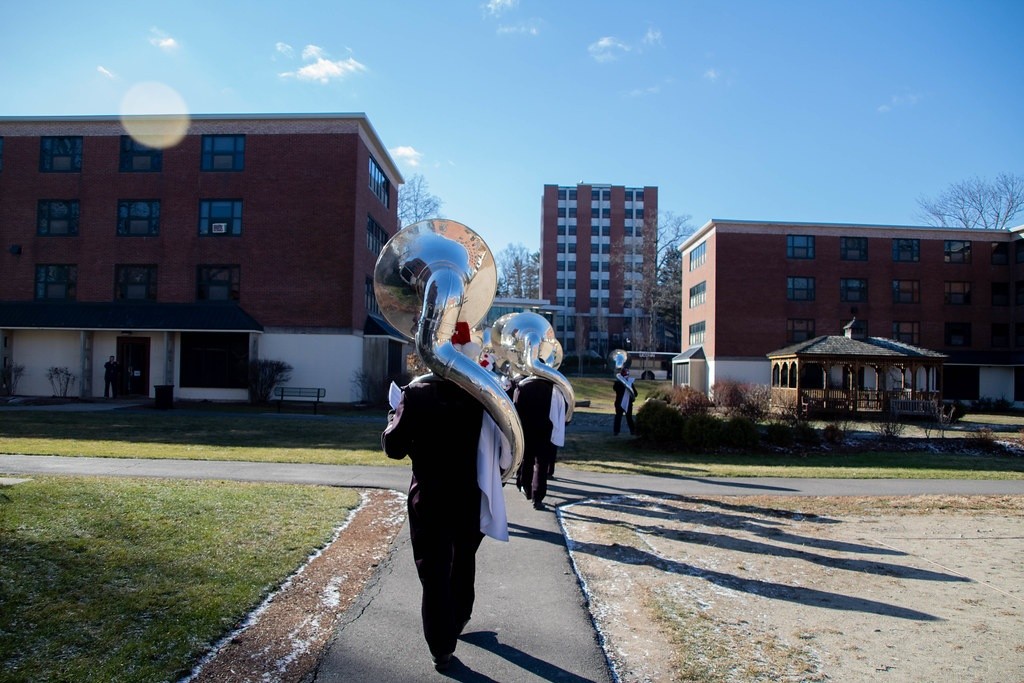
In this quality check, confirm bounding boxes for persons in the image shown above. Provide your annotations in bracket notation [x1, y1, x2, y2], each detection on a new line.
[104, 356, 120, 400]
[480, 354, 572, 510]
[614, 368, 638, 436]
[381, 321, 514, 670]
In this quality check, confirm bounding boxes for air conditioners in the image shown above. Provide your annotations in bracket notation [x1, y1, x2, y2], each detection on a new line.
[212, 223, 227, 233]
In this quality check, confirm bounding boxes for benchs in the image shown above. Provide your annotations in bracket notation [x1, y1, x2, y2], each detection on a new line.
[272, 387, 326, 415]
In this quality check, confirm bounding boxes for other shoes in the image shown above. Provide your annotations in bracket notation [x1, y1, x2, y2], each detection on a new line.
[613, 433, 618, 436]
[547, 475, 555, 480]
[534, 501, 545, 509]
[526, 494, 532, 500]
[432, 652, 452, 664]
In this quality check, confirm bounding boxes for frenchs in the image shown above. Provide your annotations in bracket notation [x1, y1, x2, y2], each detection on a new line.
[607, 348, 636, 401]
[372, 217, 526, 485]
[499, 311, 576, 424]
[489, 311, 564, 387]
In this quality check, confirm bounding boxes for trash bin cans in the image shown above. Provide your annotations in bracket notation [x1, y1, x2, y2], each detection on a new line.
[154, 384, 174, 409]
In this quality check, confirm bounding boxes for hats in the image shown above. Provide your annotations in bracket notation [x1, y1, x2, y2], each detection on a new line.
[621, 368, 629, 377]
[482, 353, 490, 360]
[451, 322, 480, 360]
[479, 359, 492, 371]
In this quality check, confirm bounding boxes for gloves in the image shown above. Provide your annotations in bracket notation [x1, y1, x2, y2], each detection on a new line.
[388, 409, 397, 421]
